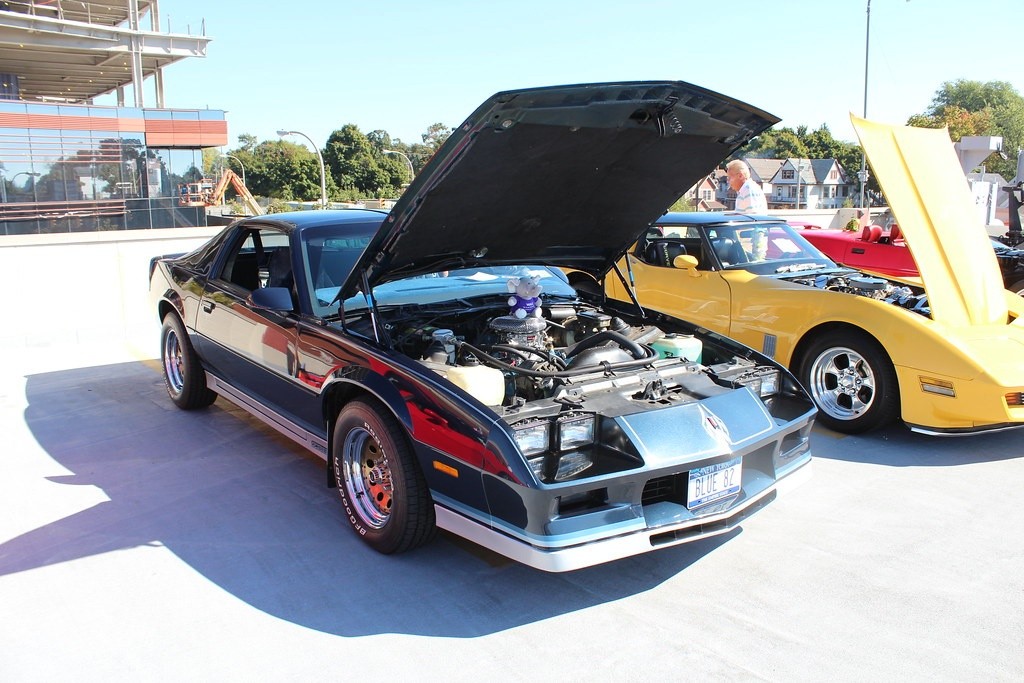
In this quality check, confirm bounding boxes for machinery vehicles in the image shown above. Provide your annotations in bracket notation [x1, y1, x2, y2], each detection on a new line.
[177, 168, 265, 216]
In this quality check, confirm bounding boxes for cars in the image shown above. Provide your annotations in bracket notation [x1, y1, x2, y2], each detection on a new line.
[146, 79, 820, 577]
[560, 110, 1024, 438]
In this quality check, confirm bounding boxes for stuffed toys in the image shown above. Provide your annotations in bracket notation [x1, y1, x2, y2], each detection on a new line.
[507, 275, 543, 318]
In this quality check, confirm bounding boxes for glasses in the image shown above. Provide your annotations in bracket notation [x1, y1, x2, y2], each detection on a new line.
[726, 174, 740, 180]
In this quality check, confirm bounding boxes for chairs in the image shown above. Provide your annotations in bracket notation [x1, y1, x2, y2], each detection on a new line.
[266, 247, 336, 315]
[703, 237, 741, 271]
[648, 242, 688, 269]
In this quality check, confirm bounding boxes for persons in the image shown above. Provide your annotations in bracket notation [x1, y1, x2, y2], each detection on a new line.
[725, 159, 768, 217]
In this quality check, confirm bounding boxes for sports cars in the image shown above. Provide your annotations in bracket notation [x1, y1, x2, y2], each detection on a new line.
[765, 217, 1024, 300]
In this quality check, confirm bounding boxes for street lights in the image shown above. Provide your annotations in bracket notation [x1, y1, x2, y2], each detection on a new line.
[277, 130, 327, 209]
[219, 154, 247, 216]
[857, 0, 913, 209]
[382, 149, 415, 181]
[11, 170, 41, 184]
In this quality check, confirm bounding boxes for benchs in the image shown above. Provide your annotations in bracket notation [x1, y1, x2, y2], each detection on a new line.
[232, 247, 368, 302]
[643, 237, 711, 270]
